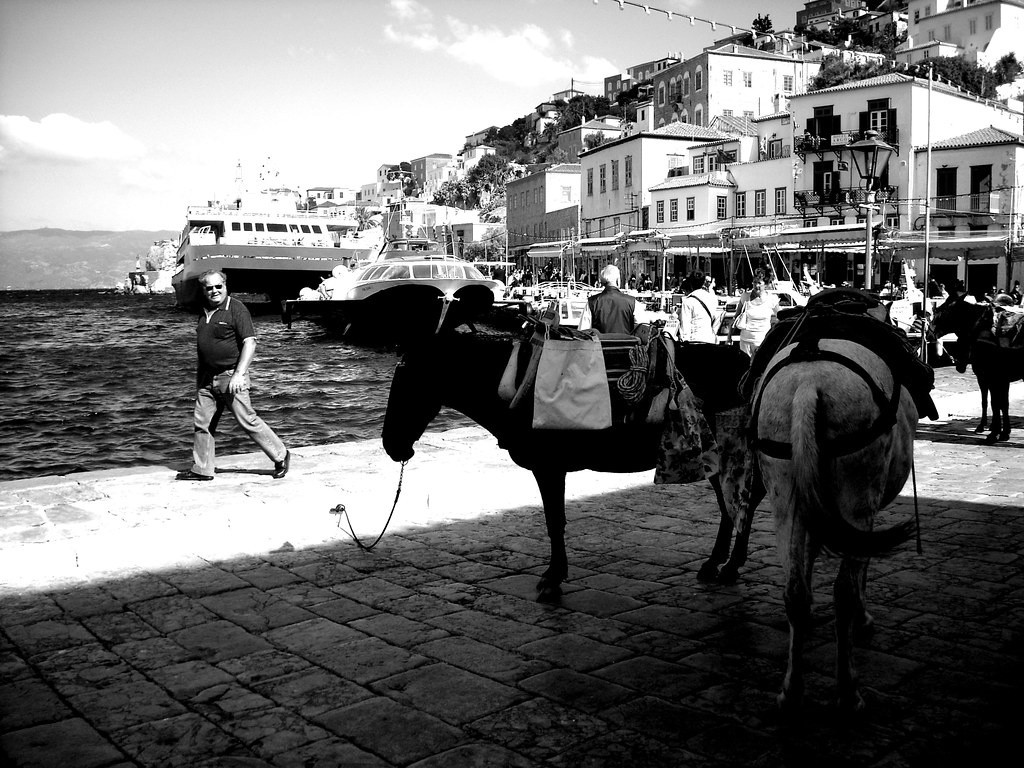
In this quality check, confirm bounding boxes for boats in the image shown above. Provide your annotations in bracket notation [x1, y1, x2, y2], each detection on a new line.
[124, 172, 497, 325]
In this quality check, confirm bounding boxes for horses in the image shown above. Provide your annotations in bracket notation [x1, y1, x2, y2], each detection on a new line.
[750, 286, 918, 728]
[382, 330, 770, 604]
[925, 290, 1024, 445]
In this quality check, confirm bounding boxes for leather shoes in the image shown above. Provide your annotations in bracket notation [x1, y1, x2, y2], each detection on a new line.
[177, 471, 214, 481]
[273, 450, 290, 478]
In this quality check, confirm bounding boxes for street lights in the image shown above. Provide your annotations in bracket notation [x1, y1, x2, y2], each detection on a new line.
[847, 129, 895, 289]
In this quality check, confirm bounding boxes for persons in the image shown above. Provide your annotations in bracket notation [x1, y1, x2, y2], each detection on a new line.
[458, 237, 464, 259]
[676, 266, 783, 367]
[577, 265, 650, 335]
[802, 129, 814, 145]
[175, 270, 291, 481]
[795, 268, 1024, 305]
[471, 258, 678, 300]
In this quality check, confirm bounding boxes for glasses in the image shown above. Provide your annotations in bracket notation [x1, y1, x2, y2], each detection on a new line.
[204, 283, 224, 290]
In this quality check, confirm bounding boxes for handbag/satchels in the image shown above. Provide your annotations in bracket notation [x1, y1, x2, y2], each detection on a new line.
[712, 405, 761, 532]
[529, 332, 614, 430]
[652, 376, 720, 484]
[735, 300, 748, 330]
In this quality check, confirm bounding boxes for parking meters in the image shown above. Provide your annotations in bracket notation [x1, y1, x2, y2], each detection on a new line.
[660, 235, 672, 311]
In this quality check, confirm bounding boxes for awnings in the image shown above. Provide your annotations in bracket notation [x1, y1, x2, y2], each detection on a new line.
[509, 239, 572, 258]
[880, 236, 1009, 262]
[664, 246, 731, 255]
[761, 240, 878, 255]
[578, 244, 621, 251]
